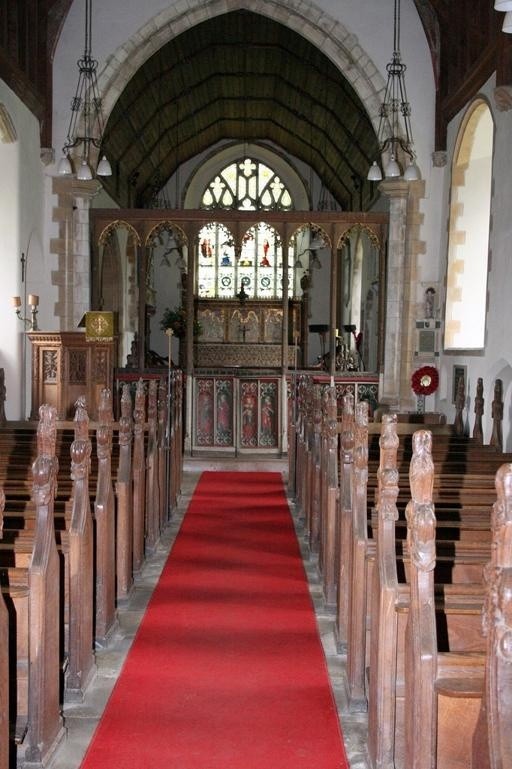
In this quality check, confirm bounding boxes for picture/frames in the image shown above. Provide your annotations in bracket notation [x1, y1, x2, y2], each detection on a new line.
[452, 365, 468, 404]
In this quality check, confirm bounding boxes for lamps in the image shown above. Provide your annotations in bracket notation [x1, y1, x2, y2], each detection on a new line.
[366, 0, 421, 181]
[58, 0, 113, 181]
[13, 295, 43, 330]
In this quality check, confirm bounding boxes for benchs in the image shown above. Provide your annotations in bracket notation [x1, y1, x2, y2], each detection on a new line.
[0, 368, 186, 769]
[282, 371, 511, 768]
